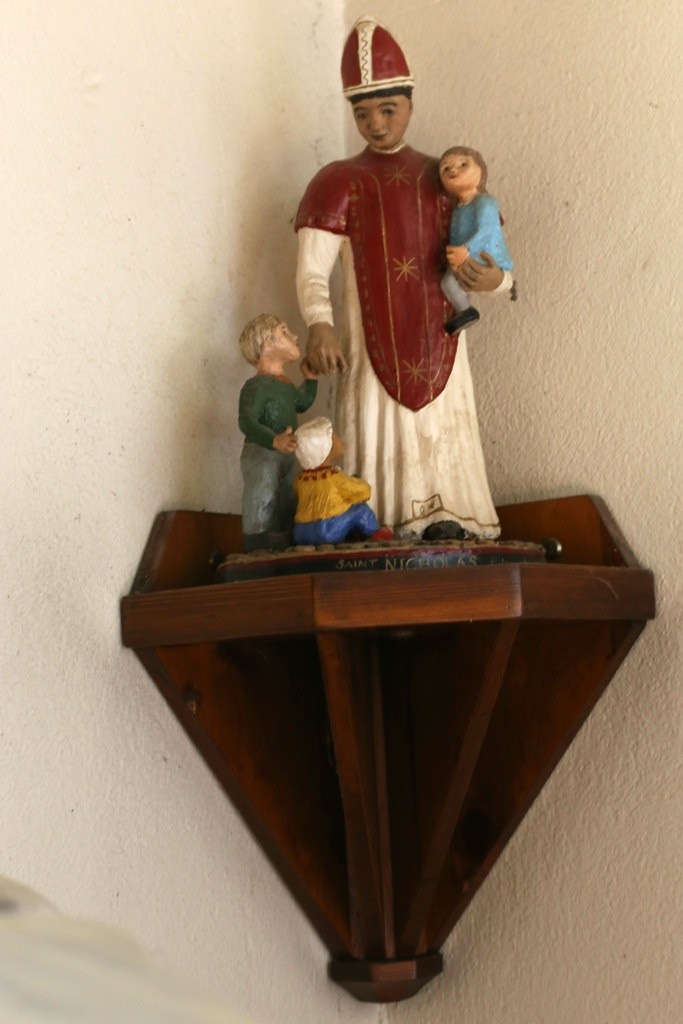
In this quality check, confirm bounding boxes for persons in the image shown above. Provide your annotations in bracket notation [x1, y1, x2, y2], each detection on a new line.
[238, 314, 319, 551]
[293, 14, 502, 542]
[437, 146, 514, 335]
[292, 415, 394, 545]
